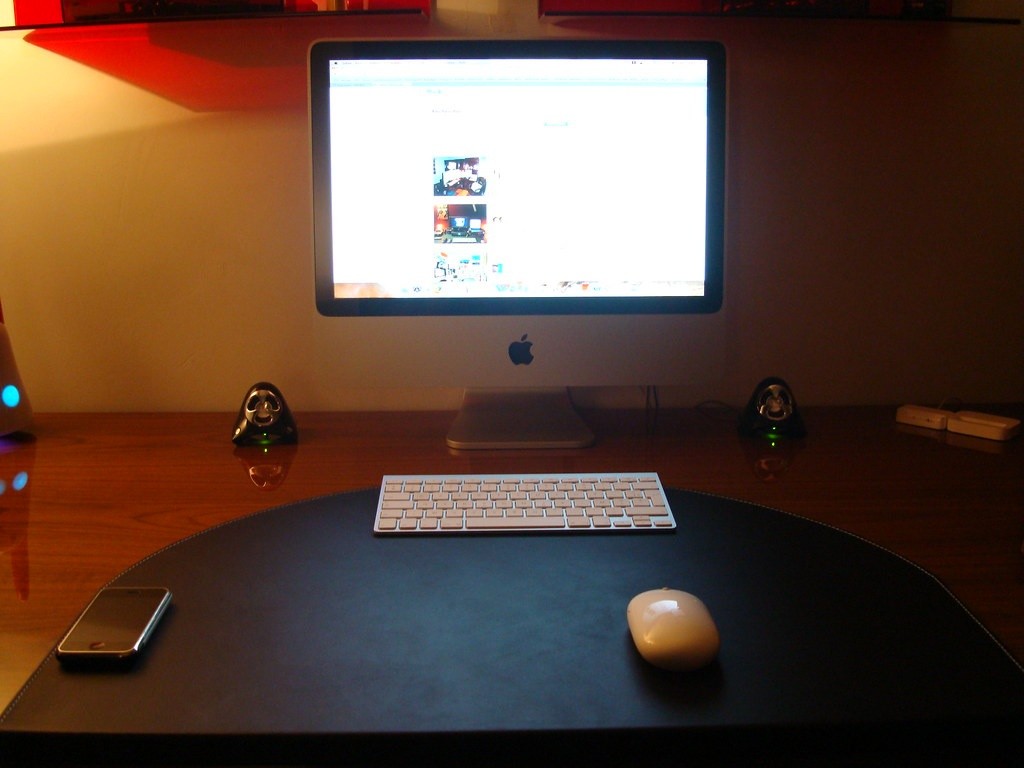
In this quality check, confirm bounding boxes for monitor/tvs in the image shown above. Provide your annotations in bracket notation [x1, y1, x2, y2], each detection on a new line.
[306, 37, 732, 450]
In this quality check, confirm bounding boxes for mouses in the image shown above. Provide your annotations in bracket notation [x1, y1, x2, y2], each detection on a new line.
[626, 587, 721, 669]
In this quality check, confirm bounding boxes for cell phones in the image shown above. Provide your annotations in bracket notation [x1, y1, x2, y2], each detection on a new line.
[54, 584, 173, 671]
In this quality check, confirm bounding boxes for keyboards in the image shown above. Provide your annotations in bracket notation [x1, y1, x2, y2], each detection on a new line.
[371, 473, 678, 531]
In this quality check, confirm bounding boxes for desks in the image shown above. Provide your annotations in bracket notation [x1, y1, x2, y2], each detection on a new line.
[0, 402, 1024, 768]
